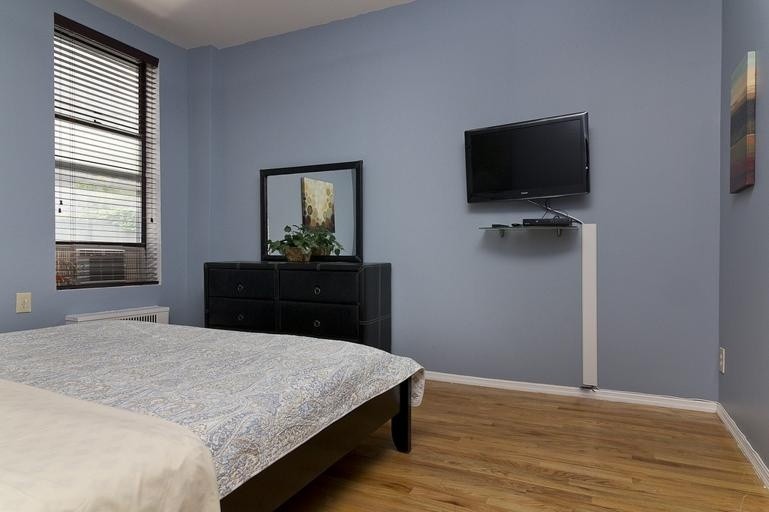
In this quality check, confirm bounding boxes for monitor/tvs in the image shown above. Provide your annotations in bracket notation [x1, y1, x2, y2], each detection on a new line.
[464, 110, 591, 203]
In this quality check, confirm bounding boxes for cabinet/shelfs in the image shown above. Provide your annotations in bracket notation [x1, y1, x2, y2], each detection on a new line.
[203, 262, 390, 353]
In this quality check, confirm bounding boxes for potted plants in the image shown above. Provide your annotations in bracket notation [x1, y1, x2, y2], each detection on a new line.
[265, 224, 320, 262]
[293, 221, 345, 262]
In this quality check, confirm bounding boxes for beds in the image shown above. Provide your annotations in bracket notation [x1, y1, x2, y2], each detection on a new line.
[0, 319, 411, 511]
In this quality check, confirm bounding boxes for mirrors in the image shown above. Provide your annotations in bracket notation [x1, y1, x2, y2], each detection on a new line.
[260, 160, 363, 262]
